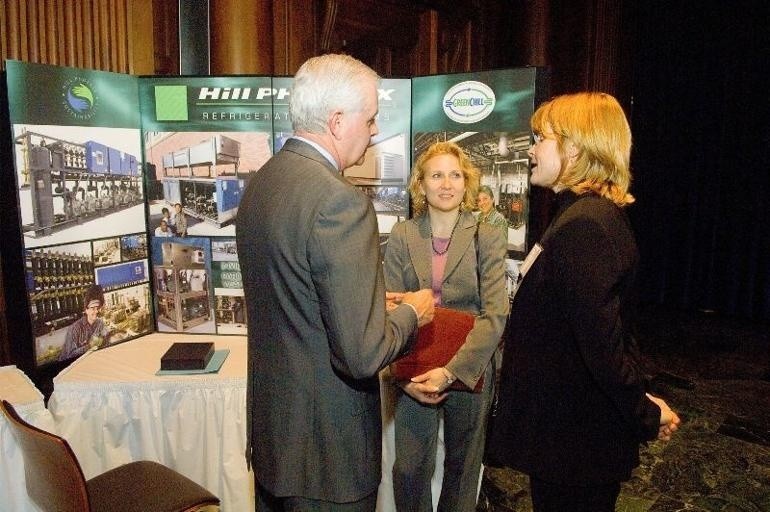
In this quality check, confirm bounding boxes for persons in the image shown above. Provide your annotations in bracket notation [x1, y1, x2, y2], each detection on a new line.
[154, 219, 172, 238]
[472, 185, 509, 234]
[235, 54, 436, 512]
[494, 91, 679, 511]
[160, 208, 171, 225]
[381, 142, 508, 512]
[57, 287, 106, 360]
[172, 204, 188, 236]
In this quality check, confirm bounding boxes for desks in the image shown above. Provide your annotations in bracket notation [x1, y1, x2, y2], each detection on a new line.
[46, 331, 487, 512]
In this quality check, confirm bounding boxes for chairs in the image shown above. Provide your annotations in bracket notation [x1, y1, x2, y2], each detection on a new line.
[2, 398, 222, 512]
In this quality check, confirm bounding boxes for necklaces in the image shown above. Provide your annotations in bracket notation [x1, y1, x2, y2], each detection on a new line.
[428, 211, 462, 255]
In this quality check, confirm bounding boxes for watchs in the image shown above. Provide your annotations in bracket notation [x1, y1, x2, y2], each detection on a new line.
[442, 369, 456, 385]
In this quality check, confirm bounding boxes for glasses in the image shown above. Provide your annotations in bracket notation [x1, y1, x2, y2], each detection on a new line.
[533, 132, 562, 143]
